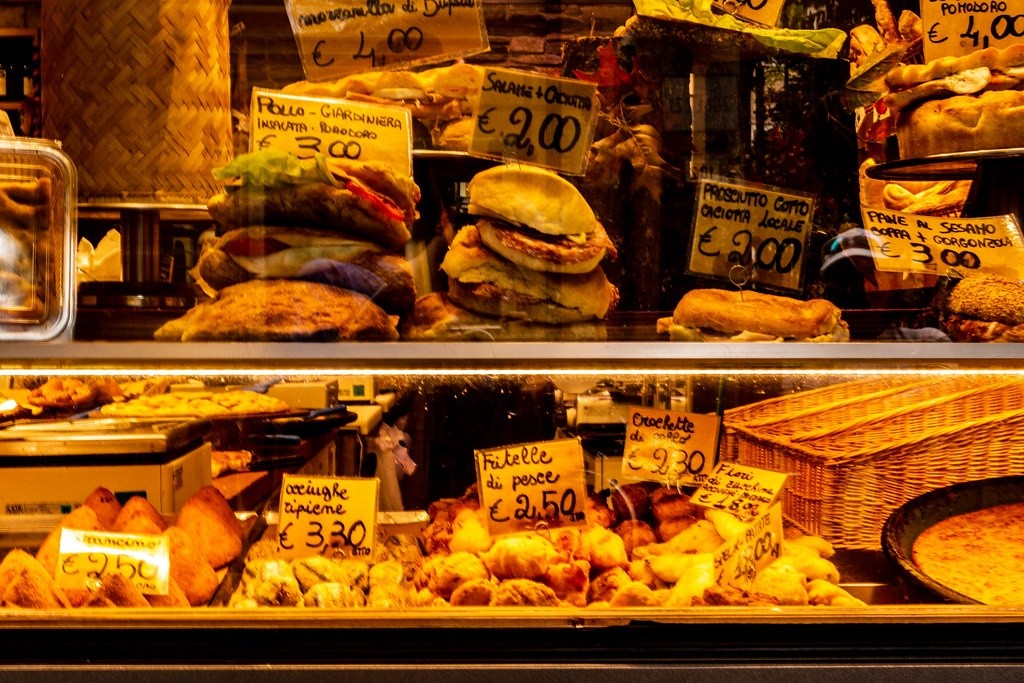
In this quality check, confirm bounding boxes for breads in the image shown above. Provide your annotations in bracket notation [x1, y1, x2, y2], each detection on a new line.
[153, 0, 1024, 342]
[0, 484, 245, 610]
[246, 475, 865, 608]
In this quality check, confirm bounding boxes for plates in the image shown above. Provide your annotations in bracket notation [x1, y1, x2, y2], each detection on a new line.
[880, 476, 1024, 605]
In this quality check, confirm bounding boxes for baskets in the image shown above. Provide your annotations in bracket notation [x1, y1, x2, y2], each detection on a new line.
[704, 374, 1023, 554]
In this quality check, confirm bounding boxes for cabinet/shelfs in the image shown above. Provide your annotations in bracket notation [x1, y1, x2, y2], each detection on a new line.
[0, 29, 39, 111]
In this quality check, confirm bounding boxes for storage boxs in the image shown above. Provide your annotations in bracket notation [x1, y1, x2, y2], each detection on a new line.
[717, 374, 1024, 552]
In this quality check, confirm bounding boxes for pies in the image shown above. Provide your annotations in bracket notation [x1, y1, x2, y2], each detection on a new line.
[913, 500, 1024, 611]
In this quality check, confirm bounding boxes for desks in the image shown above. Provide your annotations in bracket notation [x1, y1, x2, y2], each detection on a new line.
[339, 385, 426, 513]
[212, 427, 342, 514]
